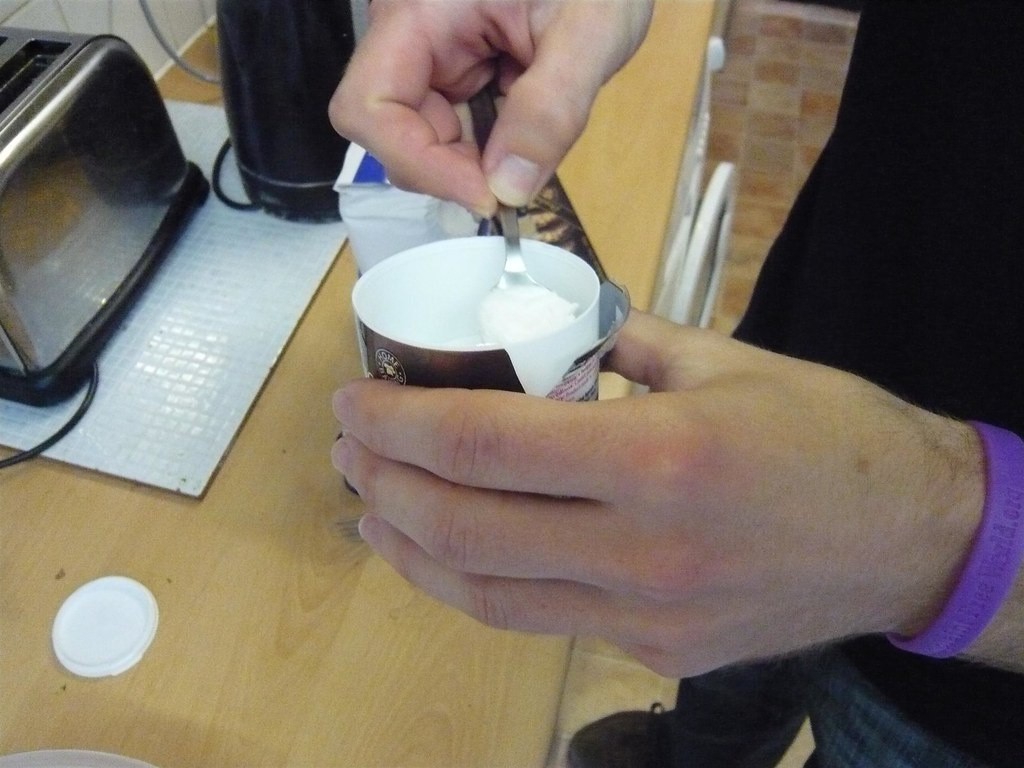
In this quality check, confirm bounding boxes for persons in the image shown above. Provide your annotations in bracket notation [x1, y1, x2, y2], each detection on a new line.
[328, 0, 1024, 767]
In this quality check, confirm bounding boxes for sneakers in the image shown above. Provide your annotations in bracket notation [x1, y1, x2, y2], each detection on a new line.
[571, 703, 713, 768]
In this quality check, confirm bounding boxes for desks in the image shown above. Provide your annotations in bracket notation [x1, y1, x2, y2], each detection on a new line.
[1, 1, 734, 768]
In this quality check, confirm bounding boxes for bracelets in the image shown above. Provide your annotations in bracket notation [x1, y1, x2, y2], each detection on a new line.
[895, 419, 1024, 659]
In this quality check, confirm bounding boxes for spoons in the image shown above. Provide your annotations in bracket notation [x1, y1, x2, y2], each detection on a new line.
[466, 81, 574, 343]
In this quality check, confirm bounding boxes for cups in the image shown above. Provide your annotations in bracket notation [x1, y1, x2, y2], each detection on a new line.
[346, 231, 605, 424]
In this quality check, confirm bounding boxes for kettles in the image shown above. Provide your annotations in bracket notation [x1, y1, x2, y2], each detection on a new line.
[212, 1, 372, 228]
